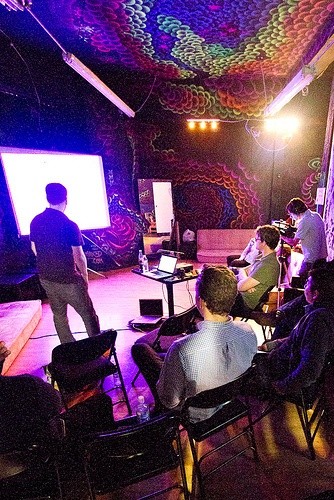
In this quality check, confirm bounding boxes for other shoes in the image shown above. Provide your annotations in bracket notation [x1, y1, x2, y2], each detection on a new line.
[148, 402, 167, 419]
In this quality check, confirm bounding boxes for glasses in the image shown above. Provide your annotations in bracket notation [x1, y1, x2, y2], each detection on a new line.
[255, 238, 263, 242]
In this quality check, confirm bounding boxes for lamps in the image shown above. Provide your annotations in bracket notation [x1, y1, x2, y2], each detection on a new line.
[262, 64, 317, 119]
[62, 52, 135, 118]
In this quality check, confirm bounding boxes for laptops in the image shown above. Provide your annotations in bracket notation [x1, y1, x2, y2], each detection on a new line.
[141, 253, 178, 280]
[131, 299, 163, 324]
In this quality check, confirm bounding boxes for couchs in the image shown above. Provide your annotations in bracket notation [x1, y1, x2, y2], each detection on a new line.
[197, 229, 255, 263]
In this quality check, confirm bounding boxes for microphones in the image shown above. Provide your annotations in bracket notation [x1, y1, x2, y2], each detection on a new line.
[158, 249, 184, 256]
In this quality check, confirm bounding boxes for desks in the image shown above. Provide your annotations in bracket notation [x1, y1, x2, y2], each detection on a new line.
[131, 264, 200, 317]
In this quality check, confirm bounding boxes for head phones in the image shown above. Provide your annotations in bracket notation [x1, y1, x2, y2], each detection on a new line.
[20, 373, 60, 468]
[289, 199, 300, 215]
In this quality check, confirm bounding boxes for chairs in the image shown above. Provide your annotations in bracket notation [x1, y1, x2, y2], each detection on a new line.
[229, 284, 276, 322]
[178, 362, 262, 497]
[246, 351, 334, 461]
[75, 407, 189, 499]
[0, 445, 58, 499]
[130, 304, 198, 386]
[47, 329, 131, 417]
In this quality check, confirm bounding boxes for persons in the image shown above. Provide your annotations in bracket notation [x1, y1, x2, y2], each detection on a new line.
[29, 183, 115, 357]
[189, 217, 296, 319]
[286, 197, 328, 289]
[0, 341, 65, 500]
[130, 265, 258, 415]
[272, 259, 334, 339]
[237, 267, 334, 399]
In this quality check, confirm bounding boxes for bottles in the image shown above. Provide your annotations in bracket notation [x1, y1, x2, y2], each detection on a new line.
[137, 395, 150, 424]
[143, 255, 148, 272]
[138, 250, 143, 271]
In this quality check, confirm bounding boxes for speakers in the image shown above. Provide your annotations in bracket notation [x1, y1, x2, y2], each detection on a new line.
[18, 268, 48, 299]
[0, 273, 40, 303]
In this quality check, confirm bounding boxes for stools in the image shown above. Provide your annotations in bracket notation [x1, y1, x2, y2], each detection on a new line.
[1, 273, 44, 303]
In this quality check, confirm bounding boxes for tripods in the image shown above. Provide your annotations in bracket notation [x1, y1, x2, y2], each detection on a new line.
[275, 236, 293, 309]
